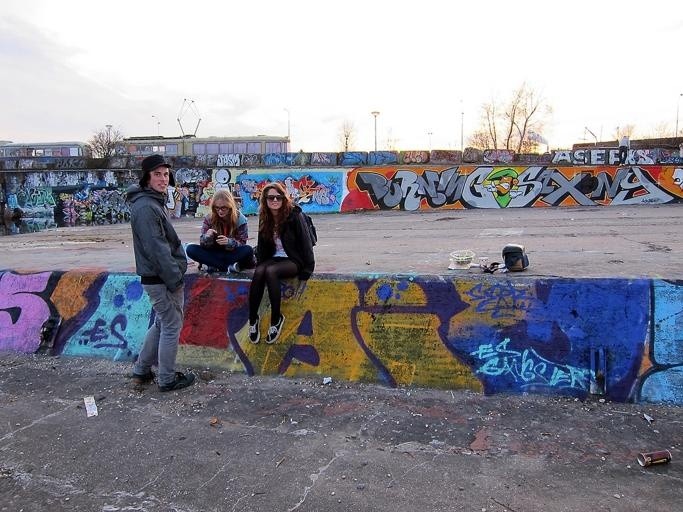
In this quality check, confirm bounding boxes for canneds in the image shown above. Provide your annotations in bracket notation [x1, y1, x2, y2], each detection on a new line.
[637, 450, 672, 466]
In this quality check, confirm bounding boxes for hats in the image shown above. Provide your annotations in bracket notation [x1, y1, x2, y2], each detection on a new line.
[141, 155, 171, 173]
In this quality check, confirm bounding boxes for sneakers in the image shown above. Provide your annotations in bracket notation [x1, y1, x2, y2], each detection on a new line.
[265, 314, 285, 344]
[248, 315, 260, 345]
[200, 263, 216, 272]
[132, 370, 154, 384]
[227, 262, 241, 273]
[159, 372, 195, 393]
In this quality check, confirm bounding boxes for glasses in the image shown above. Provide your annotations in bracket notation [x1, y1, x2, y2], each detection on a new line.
[212, 206, 229, 211]
[266, 196, 283, 200]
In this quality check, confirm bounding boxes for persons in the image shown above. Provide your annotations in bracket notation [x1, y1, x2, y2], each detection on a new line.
[186, 187, 254, 276]
[123, 153, 197, 392]
[618, 136, 630, 168]
[248, 181, 316, 344]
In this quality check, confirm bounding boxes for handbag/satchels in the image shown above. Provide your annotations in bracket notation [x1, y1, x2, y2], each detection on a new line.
[302, 214, 317, 246]
[502, 244, 529, 272]
